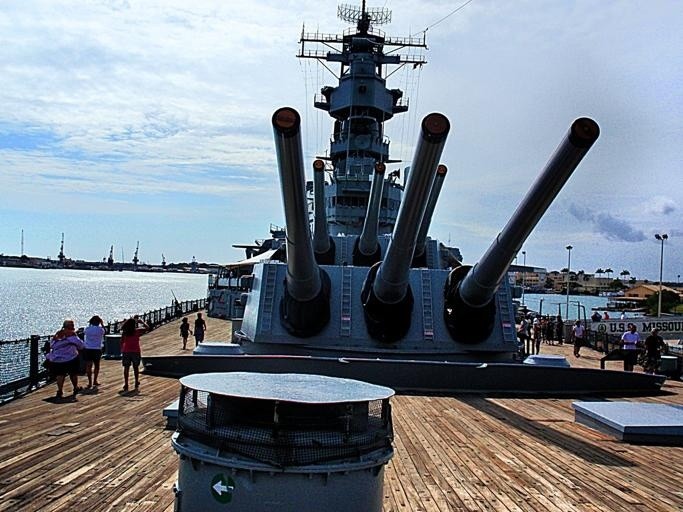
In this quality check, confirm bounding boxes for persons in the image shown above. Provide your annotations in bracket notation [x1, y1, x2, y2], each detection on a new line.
[621, 311, 628, 320]
[194, 313, 207, 346]
[84, 316, 107, 389]
[180, 318, 192, 350]
[517, 312, 564, 356]
[645, 328, 666, 374]
[622, 325, 641, 371]
[119, 318, 150, 391]
[602, 312, 609, 320]
[46, 320, 85, 399]
[591, 312, 601, 322]
[573, 319, 585, 358]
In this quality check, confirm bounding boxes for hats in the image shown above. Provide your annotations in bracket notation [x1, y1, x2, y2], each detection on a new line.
[574, 320, 582, 325]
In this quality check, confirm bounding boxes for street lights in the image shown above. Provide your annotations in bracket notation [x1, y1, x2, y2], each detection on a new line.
[522, 251, 526, 305]
[655, 234, 668, 317]
[565, 246, 573, 319]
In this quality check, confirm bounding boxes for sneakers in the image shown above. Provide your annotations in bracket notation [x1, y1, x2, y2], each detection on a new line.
[56, 391, 63, 398]
[72, 392, 77, 400]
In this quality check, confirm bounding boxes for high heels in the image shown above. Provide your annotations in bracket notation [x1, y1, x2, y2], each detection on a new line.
[135, 382, 140, 387]
[123, 385, 128, 391]
